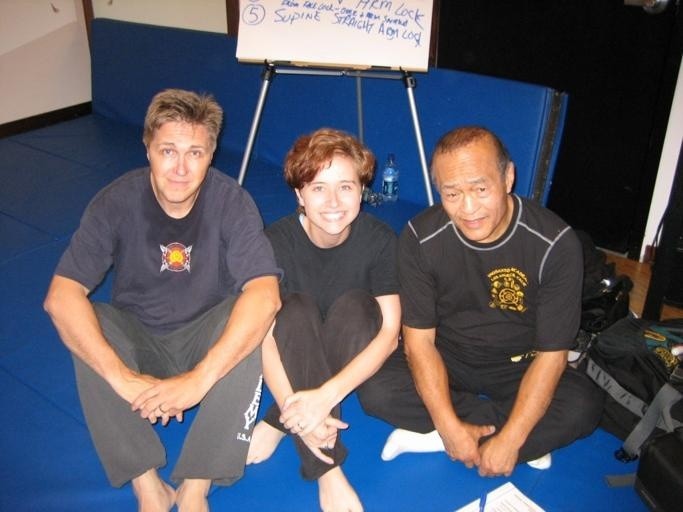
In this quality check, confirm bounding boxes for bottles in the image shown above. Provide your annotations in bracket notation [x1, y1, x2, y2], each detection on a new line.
[382, 153, 399, 202]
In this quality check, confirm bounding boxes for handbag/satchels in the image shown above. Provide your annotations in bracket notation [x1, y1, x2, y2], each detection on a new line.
[577, 229, 683, 455]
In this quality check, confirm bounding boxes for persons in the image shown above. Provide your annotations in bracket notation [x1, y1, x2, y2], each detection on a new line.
[357, 125, 603, 480]
[242, 129, 406, 510]
[41, 86, 288, 510]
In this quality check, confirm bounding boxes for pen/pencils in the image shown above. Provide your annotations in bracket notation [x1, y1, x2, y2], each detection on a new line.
[479, 489, 487, 512]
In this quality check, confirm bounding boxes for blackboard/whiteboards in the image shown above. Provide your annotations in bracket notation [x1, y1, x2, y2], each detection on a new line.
[236, 0, 440, 72]
